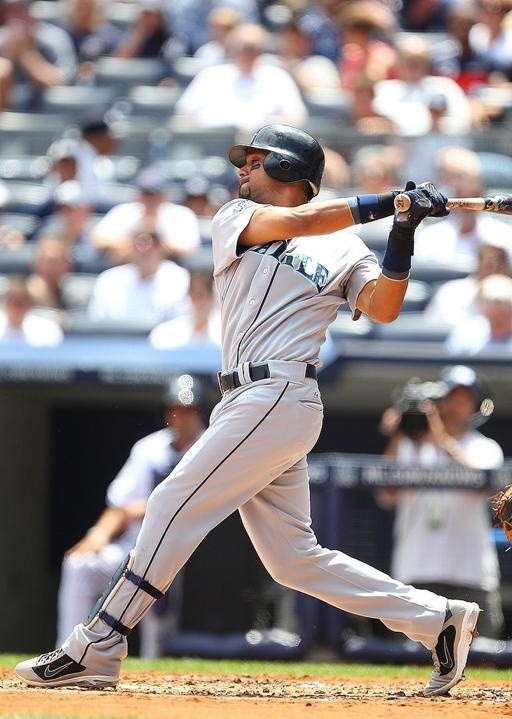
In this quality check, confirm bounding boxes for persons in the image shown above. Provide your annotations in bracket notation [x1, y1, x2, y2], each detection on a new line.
[50, 372, 223, 654]
[15, 126, 482, 690]
[375, 363, 505, 641]
[0, 0, 511, 362]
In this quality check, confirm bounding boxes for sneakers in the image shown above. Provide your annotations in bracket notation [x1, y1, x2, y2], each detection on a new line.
[14, 649, 119, 687]
[423, 598, 480, 698]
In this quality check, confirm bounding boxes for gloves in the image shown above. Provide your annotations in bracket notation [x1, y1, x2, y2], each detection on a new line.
[382, 181, 435, 270]
[357, 180, 449, 223]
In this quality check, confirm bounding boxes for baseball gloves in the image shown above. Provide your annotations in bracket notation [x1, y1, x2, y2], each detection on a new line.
[493, 484, 512, 541]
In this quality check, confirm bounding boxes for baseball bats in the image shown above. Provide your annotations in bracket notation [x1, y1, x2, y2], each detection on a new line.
[394, 193, 512, 215]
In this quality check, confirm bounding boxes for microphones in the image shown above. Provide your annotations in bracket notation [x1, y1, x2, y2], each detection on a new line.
[446, 419, 475, 429]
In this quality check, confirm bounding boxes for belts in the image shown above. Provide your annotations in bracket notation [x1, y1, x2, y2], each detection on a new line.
[217, 364, 317, 391]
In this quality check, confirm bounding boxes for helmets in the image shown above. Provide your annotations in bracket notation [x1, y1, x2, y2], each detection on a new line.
[229, 124, 325, 197]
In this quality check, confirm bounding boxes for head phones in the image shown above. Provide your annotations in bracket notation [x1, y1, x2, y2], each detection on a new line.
[471, 409, 493, 431]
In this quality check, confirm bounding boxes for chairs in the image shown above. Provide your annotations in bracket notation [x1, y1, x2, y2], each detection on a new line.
[0, 0, 512, 347]
